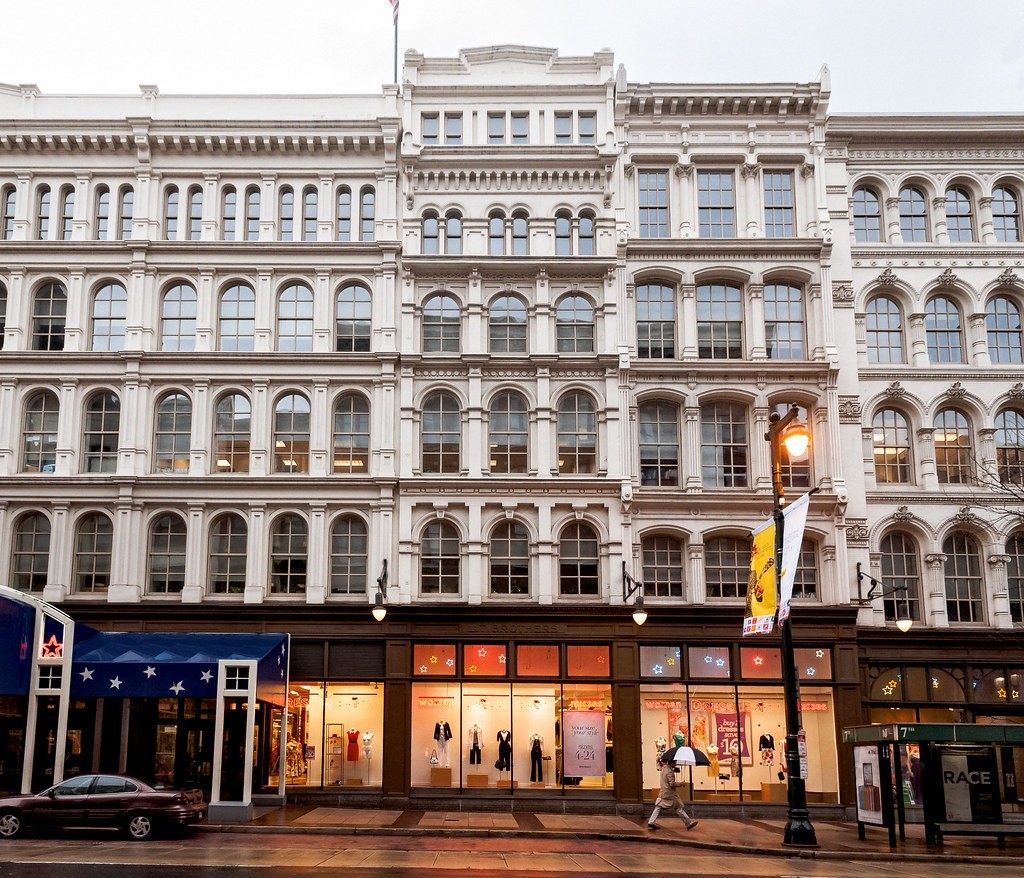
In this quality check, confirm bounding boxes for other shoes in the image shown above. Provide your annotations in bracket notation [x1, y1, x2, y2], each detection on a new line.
[687, 822, 698, 830]
[648, 824, 660, 829]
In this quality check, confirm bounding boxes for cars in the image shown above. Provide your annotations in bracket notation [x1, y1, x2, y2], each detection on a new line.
[0, 774, 210, 842]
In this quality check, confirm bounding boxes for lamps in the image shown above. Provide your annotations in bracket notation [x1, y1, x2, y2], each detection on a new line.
[858, 562, 913, 633]
[372, 558, 387, 621]
[622, 560, 647, 626]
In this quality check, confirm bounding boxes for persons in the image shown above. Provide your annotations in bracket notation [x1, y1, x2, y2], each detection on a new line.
[469, 723, 483, 764]
[347, 729, 360, 762]
[647, 760, 698, 831]
[529, 733, 544, 782]
[729, 742, 740, 776]
[497, 728, 511, 772]
[759, 733, 776, 766]
[706, 743, 720, 777]
[433, 720, 452, 767]
[362, 730, 374, 759]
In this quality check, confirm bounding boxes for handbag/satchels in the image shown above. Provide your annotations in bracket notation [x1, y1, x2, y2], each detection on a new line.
[778, 771, 785, 781]
[542, 756, 551, 760]
[430, 749, 438, 763]
[719, 773, 730, 779]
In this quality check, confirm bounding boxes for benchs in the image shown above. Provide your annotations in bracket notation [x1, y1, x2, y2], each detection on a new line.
[935, 823, 1024, 850]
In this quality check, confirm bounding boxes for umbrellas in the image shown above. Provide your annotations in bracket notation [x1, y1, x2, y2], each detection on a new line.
[659, 744, 712, 788]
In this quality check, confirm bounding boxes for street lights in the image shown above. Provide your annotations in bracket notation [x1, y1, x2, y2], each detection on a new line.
[763, 403, 810, 844]
